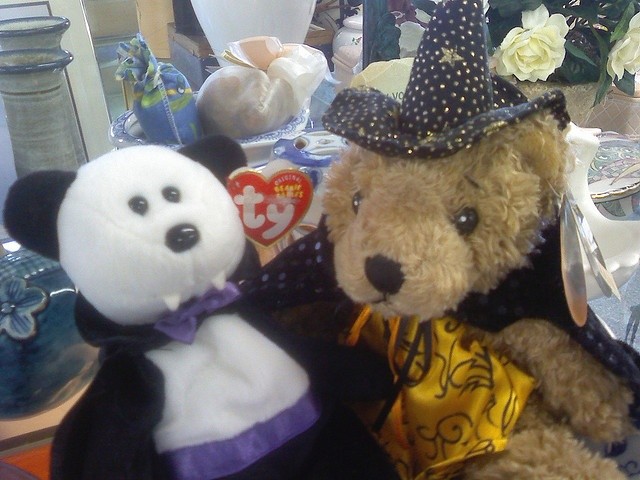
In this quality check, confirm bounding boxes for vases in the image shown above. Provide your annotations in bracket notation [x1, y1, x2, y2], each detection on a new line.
[1, 49, 79, 178]
[0, 16, 70, 50]
[107, 105, 311, 169]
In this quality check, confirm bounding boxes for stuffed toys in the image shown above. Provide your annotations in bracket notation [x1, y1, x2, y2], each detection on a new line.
[277, 0, 634, 480]
[2, 136, 397, 479]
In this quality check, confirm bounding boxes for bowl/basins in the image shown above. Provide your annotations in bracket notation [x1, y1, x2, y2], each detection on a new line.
[108, 91, 310, 170]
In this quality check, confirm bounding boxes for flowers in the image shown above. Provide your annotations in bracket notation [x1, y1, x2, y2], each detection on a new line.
[486, 0, 639, 112]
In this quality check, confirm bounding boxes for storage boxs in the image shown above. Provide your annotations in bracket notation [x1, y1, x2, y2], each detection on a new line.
[166, 21, 332, 93]
